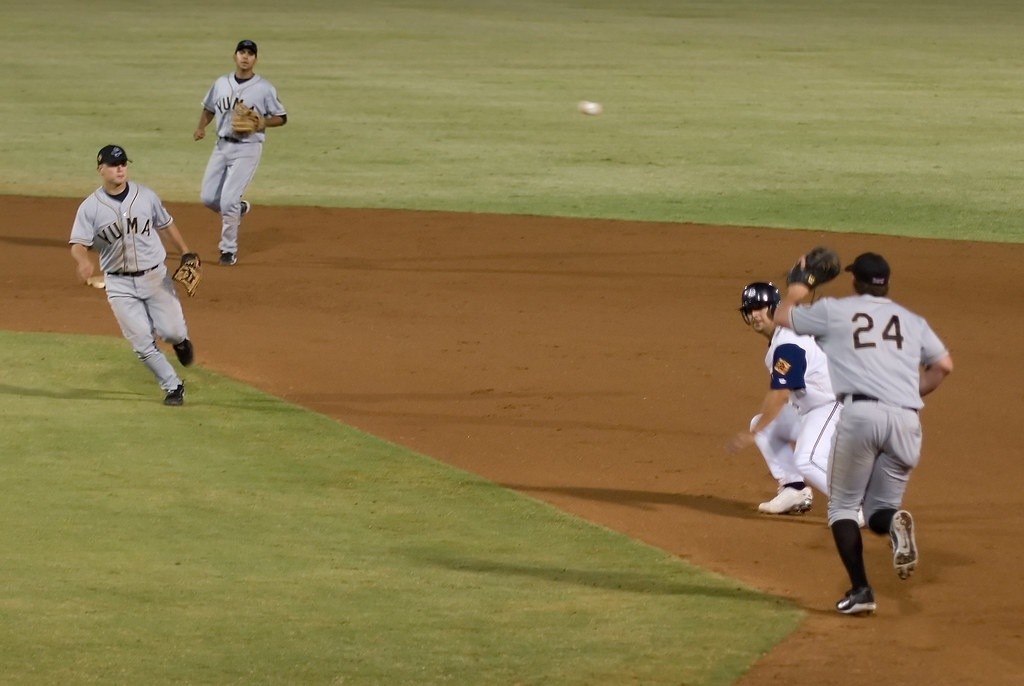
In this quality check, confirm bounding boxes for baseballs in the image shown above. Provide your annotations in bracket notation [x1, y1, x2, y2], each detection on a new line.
[585, 102, 600, 115]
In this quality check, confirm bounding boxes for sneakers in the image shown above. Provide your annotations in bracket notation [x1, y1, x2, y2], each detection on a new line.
[835, 587, 876, 614]
[856, 505, 866, 528]
[889, 509, 919, 580]
[219, 252, 237, 265]
[173, 338, 193, 366]
[241, 200, 250, 217]
[164, 380, 185, 406]
[758, 485, 813, 514]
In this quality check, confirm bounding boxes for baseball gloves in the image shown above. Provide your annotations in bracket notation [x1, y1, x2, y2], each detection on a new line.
[230, 102, 266, 136]
[786, 244, 842, 306]
[170, 252, 204, 299]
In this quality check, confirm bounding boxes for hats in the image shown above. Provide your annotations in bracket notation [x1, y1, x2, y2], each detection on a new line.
[96, 145, 127, 165]
[235, 40, 257, 54]
[845, 252, 891, 286]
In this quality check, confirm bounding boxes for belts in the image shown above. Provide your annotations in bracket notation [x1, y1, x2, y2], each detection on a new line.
[852, 394, 918, 413]
[220, 136, 239, 143]
[108, 265, 158, 276]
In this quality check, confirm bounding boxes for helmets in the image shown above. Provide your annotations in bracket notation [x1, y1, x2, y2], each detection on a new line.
[739, 282, 780, 326]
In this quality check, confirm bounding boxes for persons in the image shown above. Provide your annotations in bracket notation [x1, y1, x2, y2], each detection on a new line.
[69, 145, 202, 405]
[739, 280, 865, 528]
[194, 40, 287, 266]
[773, 244, 953, 615]
